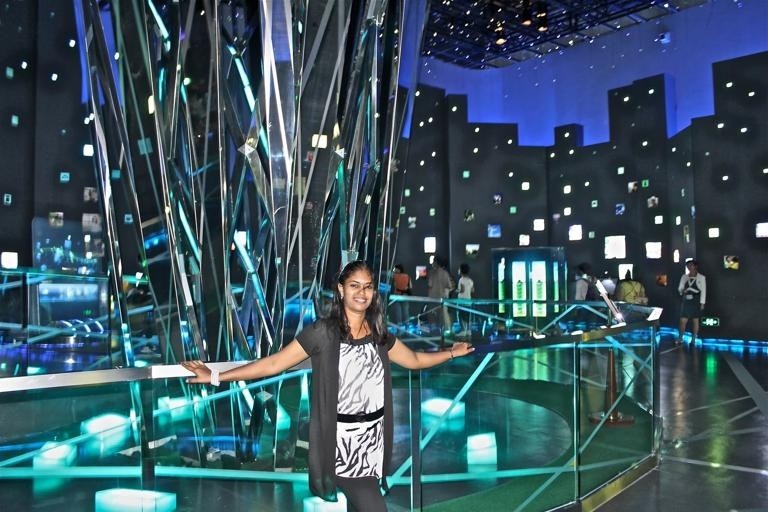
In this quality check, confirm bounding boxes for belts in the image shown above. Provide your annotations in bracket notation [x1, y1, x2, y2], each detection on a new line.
[337, 407, 384, 422]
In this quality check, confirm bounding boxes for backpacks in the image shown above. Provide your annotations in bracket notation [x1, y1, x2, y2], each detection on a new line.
[582, 277, 604, 300]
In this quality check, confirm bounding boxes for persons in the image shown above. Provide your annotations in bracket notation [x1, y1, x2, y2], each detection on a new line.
[674, 261, 707, 346]
[180, 260, 476, 511]
[568, 262, 649, 308]
[391, 264, 412, 327]
[427, 255, 452, 334]
[454, 263, 475, 337]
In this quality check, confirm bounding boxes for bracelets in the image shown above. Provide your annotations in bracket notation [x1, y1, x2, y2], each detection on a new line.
[210, 370, 220, 387]
[450, 350, 453, 359]
